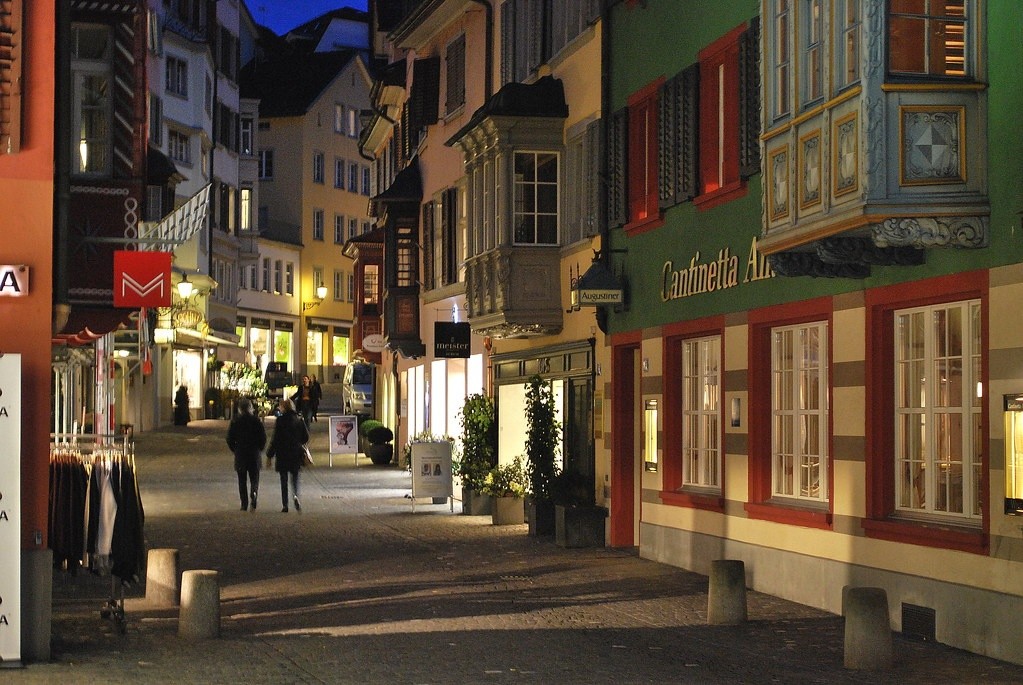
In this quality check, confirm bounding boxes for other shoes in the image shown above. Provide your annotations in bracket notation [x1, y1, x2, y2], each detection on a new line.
[281, 507, 288, 512]
[294, 495, 301, 510]
[250, 491, 257, 509]
[302, 443, 314, 467]
[240, 506, 247, 511]
[314, 417, 317, 422]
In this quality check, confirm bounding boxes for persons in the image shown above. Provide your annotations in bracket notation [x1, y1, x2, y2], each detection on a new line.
[297, 372, 323, 431]
[266, 398, 310, 513]
[226, 399, 268, 511]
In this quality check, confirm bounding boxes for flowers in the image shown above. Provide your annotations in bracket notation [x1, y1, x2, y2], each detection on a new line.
[400, 428, 461, 486]
[454, 387, 496, 498]
[480, 455, 530, 500]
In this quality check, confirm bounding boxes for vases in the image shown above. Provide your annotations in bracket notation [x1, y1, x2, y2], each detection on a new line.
[490, 495, 525, 526]
[432, 497, 447, 504]
[462, 488, 490, 516]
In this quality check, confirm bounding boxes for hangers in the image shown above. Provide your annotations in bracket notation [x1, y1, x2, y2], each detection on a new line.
[50, 441, 135, 465]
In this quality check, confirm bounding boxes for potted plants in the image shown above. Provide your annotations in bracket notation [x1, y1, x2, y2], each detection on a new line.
[549, 469, 607, 547]
[359, 420, 384, 458]
[368, 426, 393, 465]
[522, 374, 565, 536]
[174, 385, 190, 426]
[205, 354, 268, 420]
[283, 385, 299, 401]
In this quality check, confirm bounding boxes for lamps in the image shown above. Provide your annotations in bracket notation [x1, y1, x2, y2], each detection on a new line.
[154, 271, 194, 317]
[303, 282, 328, 312]
